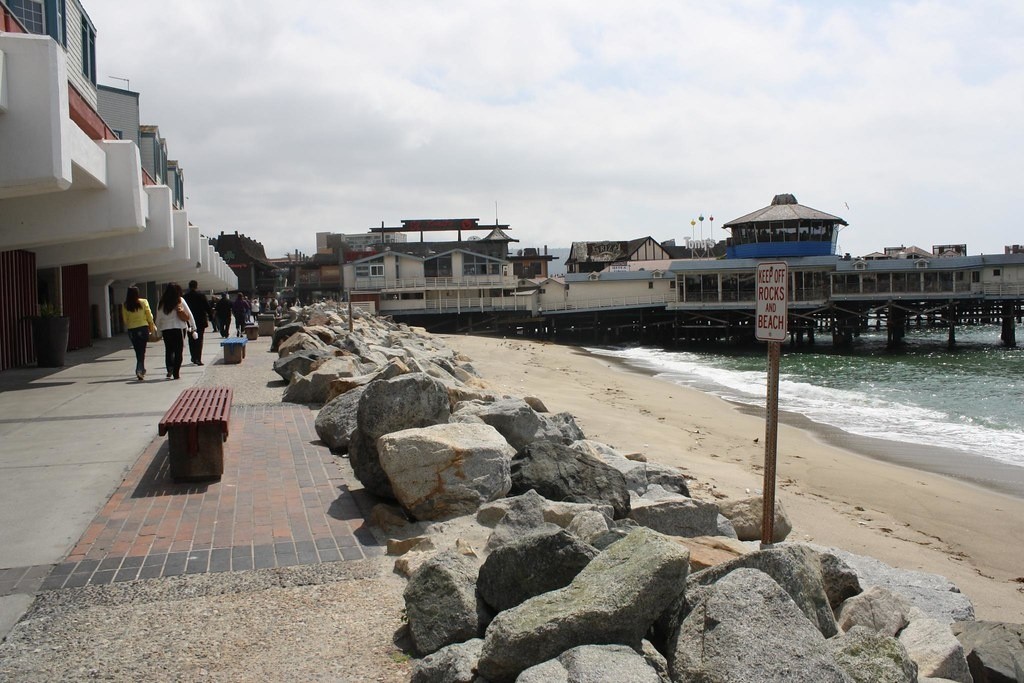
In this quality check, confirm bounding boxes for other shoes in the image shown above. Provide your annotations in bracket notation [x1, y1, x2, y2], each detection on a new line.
[167, 367, 173, 377]
[194, 359, 204, 366]
[214, 330, 243, 338]
[135, 371, 146, 381]
[174, 372, 180, 379]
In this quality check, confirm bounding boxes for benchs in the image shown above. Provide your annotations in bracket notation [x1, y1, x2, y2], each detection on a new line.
[245, 322, 259, 340]
[220, 337, 248, 364]
[158, 387, 233, 481]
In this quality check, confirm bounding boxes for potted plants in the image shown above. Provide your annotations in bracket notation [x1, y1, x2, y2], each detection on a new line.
[20, 299, 70, 368]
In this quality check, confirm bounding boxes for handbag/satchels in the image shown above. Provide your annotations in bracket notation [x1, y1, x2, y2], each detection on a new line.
[148, 325, 162, 343]
[176, 297, 190, 322]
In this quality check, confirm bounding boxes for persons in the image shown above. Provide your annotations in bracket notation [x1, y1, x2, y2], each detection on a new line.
[183, 280, 326, 366]
[157, 281, 196, 379]
[123, 285, 153, 381]
